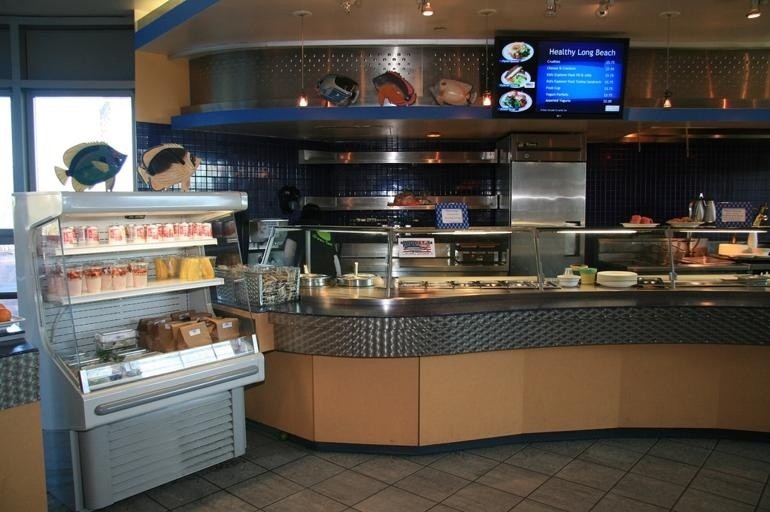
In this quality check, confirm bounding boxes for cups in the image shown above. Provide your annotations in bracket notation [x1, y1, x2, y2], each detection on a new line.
[47, 259, 149, 298]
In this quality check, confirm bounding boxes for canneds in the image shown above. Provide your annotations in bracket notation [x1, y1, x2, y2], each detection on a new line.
[61, 225, 99, 244]
[108, 222, 213, 243]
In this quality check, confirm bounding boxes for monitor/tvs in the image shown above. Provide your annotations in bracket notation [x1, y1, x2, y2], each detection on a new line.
[491, 30, 632, 120]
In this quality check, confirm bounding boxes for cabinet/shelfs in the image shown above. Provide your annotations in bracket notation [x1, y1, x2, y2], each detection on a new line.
[297, 147, 497, 211]
[15, 190, 266, 510]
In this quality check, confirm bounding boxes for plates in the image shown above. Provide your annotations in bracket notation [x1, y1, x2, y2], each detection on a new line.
[597, 270, 638, 289]
[501, 69, 532, 88]
[667, 222, 706, 229]
[621, 223, 660, 229]
[499, 91, 533, 113]
[502, 42, 534, 63]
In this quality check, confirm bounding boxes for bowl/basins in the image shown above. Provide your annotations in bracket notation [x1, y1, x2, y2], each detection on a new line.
[558, 275, 580, 288]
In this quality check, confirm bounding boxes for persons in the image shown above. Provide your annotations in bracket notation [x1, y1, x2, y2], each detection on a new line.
[281, 201, 342, 278]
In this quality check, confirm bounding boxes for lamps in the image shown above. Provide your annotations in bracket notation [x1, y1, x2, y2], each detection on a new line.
[420, 1, 436, 16]
[478, 8, 497, 107]
[294, 10, 312, 108]
[660, 11, 679, 109]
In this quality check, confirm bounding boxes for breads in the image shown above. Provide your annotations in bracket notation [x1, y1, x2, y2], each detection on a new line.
[0, 303, 11, 322]
